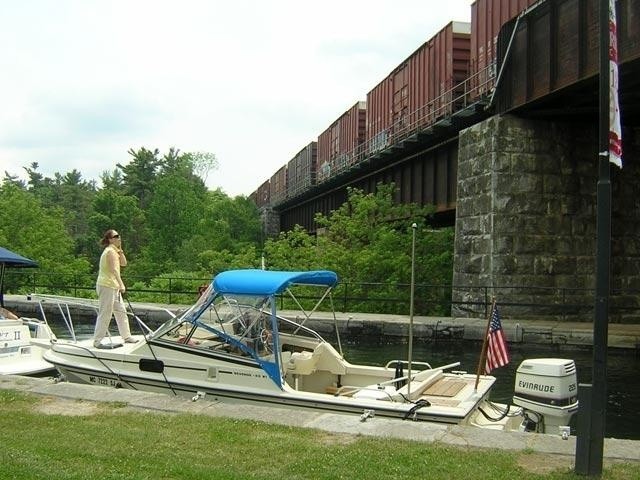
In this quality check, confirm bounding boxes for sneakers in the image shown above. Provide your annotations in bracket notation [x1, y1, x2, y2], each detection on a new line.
[94, 340, 103, 348]
[125, 337, 139, 343]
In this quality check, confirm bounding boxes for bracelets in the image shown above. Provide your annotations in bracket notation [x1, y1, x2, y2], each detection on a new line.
[118, 250, 124, 254]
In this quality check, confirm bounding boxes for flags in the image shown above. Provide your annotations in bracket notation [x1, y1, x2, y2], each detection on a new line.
[483, 305, 509, 379]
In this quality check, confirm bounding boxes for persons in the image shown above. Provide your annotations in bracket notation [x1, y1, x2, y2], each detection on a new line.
[92, 228, 140, 348]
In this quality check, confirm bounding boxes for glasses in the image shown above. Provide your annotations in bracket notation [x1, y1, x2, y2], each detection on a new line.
[111, 235, 119, 238]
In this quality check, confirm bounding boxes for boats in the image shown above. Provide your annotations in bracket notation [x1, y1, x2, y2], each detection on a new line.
[0, 247, 57, 376]
[30, 269, 578, 435]
